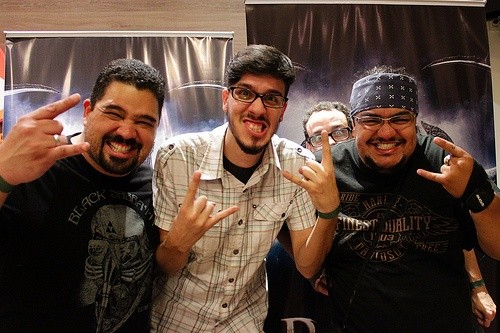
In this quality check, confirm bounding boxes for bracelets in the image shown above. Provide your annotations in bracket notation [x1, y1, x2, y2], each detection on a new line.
[469, 279, 484, 288]
[318, 202, 341, 219]
[0, 176, 17, 193]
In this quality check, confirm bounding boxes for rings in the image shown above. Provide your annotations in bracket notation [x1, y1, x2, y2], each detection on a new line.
[54, 134, 62, 147]
[444, 155, 453, 166]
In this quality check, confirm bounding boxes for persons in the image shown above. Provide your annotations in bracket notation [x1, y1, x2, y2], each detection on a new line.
[0, 59, 163, 332]
[151, 45, 341, 333]
[277, 72, 500, 333]
[305, 102, 496, 327]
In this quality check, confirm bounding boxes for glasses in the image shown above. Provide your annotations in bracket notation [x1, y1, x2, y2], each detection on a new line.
[353, 113, 417, 126]
[228, 86, 289, 109]
[306, 126, 352, 147]
